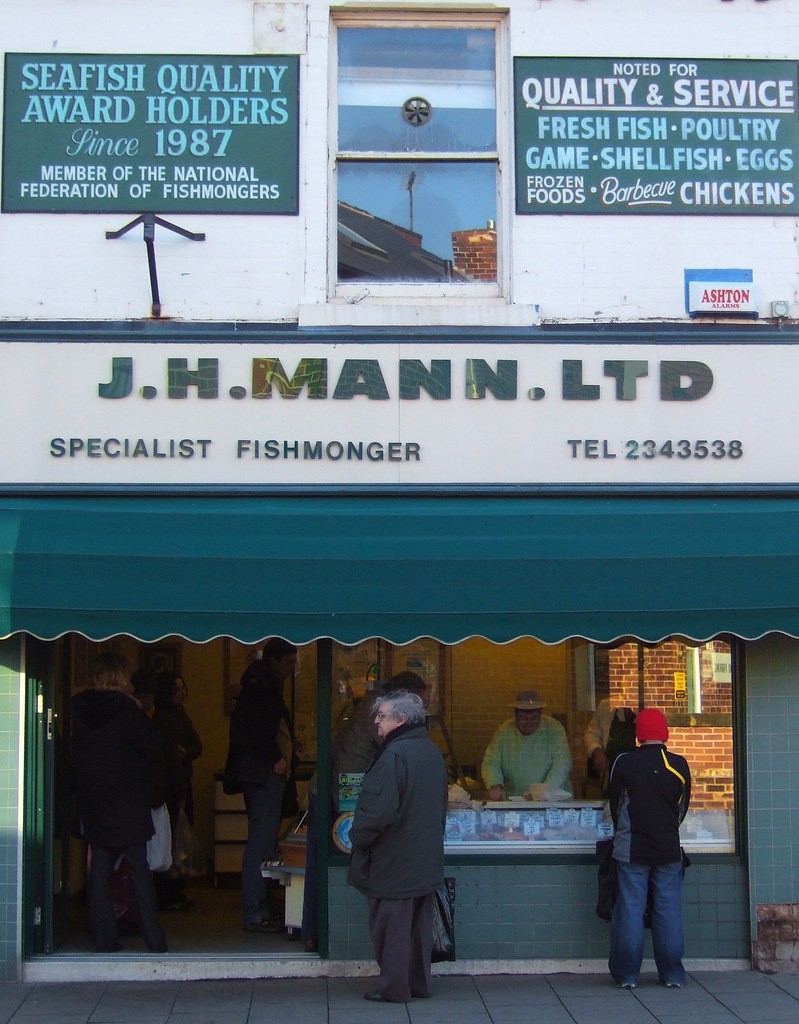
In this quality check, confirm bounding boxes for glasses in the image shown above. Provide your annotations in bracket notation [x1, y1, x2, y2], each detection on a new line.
[376, 711, 387, 718]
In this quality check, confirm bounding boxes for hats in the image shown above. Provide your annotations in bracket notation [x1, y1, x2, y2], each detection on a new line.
[130, 671, 158, 694]
[635, 708, 669, 743]
[506, 689, 548, 709]
[89, 651, 128, 673]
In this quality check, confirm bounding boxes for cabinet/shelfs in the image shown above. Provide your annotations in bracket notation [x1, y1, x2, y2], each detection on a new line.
[213, 765, 314, 889]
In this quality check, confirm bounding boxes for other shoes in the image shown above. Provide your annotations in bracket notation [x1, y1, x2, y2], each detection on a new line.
[160, 896, 196, 912]
[617, 982, 636, 989]
[243, 911, 282, 932]
[364, 990, 393, 1002]
[666, 982, 681, 988]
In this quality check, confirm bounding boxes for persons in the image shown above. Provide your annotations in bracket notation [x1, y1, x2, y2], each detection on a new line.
[480, 691, 575, 802]
[607, 706, 692, 989]
[584, 667, 660, 800]
[347, 688, 448, 1003]
[304, 670, 426, 814]
[64, 645, 202, 954]
[222, 638, 298, 933]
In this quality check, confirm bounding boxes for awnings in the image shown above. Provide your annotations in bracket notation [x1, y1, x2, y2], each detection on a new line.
[0, 495, 799, 646]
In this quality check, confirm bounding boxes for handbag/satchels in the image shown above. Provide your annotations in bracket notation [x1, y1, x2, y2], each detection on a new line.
[596, 840, 690, 929]
[171, 808, 196, 865]
[146, 802, 172, 873]
[244, 778, 299, 819]
[431, 878, 455, 963]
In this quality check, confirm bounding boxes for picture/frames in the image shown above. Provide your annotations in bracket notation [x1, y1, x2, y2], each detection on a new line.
[139, 641, 183, 683]
